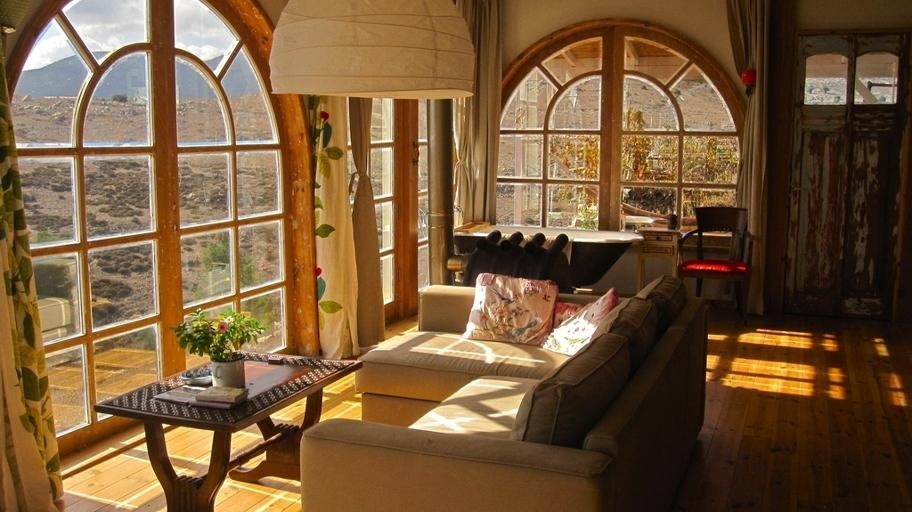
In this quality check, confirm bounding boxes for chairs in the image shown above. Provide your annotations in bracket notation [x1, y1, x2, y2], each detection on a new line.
[675, 207, 755, 328]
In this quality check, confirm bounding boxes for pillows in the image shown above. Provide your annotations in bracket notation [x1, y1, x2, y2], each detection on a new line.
[466, 272, 616, 357]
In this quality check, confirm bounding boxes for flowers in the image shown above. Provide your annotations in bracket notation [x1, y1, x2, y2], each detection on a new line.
[169, 307, 269, 361]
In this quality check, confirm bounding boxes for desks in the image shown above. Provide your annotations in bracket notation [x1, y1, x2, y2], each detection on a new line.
[454, 224, 644, 293]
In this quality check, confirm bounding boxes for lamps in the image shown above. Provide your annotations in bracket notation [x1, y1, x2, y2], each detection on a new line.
[268, 1, 476, 100]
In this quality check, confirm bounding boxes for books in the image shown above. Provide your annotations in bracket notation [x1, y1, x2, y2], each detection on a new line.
[153, 384, 248, 410]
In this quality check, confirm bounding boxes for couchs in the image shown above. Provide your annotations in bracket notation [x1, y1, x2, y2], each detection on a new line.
[300, 285, 708, 511]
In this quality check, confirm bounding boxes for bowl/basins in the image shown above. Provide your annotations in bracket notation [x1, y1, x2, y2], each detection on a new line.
[179, 368, 212, 385]
